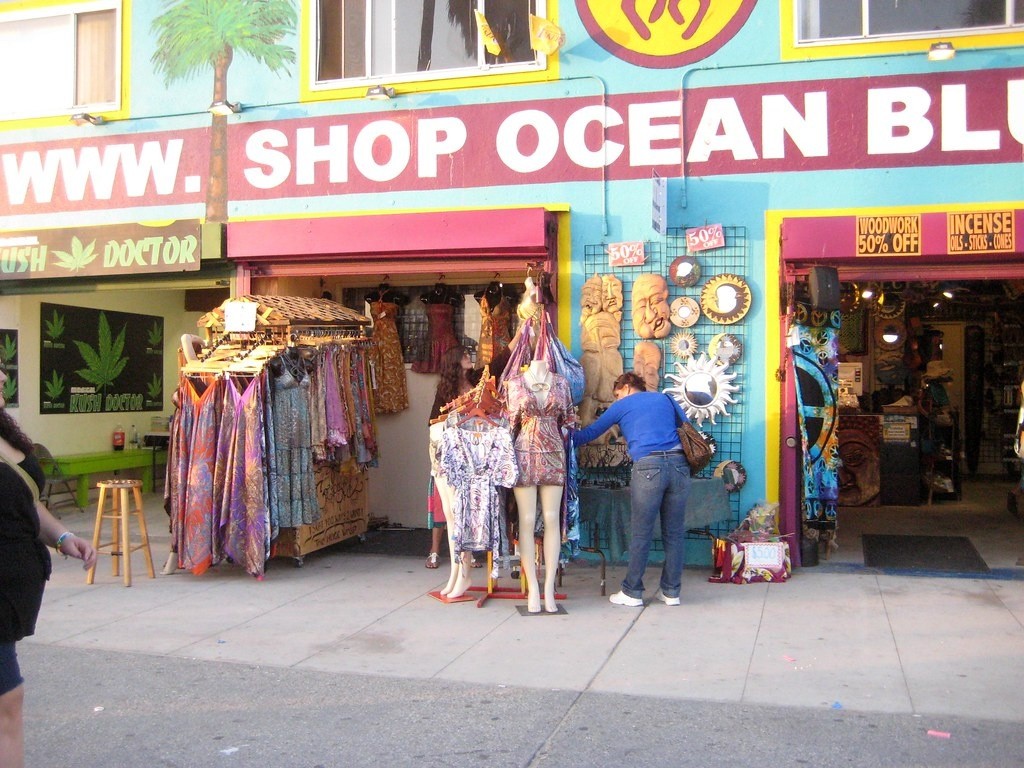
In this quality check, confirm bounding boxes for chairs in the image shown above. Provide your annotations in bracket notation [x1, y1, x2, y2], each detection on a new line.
[34, 443, 83, 519]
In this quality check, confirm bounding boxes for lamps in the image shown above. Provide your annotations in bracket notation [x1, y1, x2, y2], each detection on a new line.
[861, 281, 875, 299]
[364, 86, 391, 102]
[209, 99, 239, 115]
[927, 38, 957, 61]
[70, 113, 103, 126]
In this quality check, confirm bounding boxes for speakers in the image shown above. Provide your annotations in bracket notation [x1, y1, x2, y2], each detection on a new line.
[808, 266, 840, 310]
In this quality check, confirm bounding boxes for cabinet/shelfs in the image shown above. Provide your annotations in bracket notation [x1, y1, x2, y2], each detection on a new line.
[921, 413, 957, 502]
[998, 323, 1024, 463]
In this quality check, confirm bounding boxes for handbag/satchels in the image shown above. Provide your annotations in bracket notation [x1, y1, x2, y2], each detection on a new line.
[666, 393, 712, 476]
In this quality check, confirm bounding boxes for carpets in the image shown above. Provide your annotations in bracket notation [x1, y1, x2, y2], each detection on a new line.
[862, 533, 991, 574]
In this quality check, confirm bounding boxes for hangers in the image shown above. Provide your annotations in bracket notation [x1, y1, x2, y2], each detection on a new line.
[181, 327, 375, 378]
[431, 384, 506, 435]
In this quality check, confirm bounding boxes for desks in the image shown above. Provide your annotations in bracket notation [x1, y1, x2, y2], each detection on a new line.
[38, 449, 153, 506]
[572, 474, 728, 595]
[145, 432, 171, 487]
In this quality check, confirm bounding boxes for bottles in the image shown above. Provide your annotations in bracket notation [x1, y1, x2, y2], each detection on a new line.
[129, 424, 138, 450]
[112, 423, 125, 451]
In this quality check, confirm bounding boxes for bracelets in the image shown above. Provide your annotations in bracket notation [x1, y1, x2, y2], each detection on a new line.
[55, 532, 74, 560]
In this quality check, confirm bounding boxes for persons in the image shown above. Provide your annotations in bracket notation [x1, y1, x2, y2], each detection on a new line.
[516, 276, 541, 351]
[474, 281, 520, 370]
[568, 372, 691, 607]
[425, 328, 522, 569]
[364, 283, 411, 416]
[0, 344, 107, 768]
[429, 421, 472, 598]
[410, 283, 463, 373]
[160, 333, 207, 575]
[269, 347, 323, 528]
[507, 360, 574, 613]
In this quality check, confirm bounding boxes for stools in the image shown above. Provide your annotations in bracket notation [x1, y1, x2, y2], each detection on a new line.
[86, 480, 155, 586]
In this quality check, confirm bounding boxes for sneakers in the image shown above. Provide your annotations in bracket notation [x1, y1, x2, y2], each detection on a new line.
[609, 588, 643, 607]
[655, 588, 680, 607]
